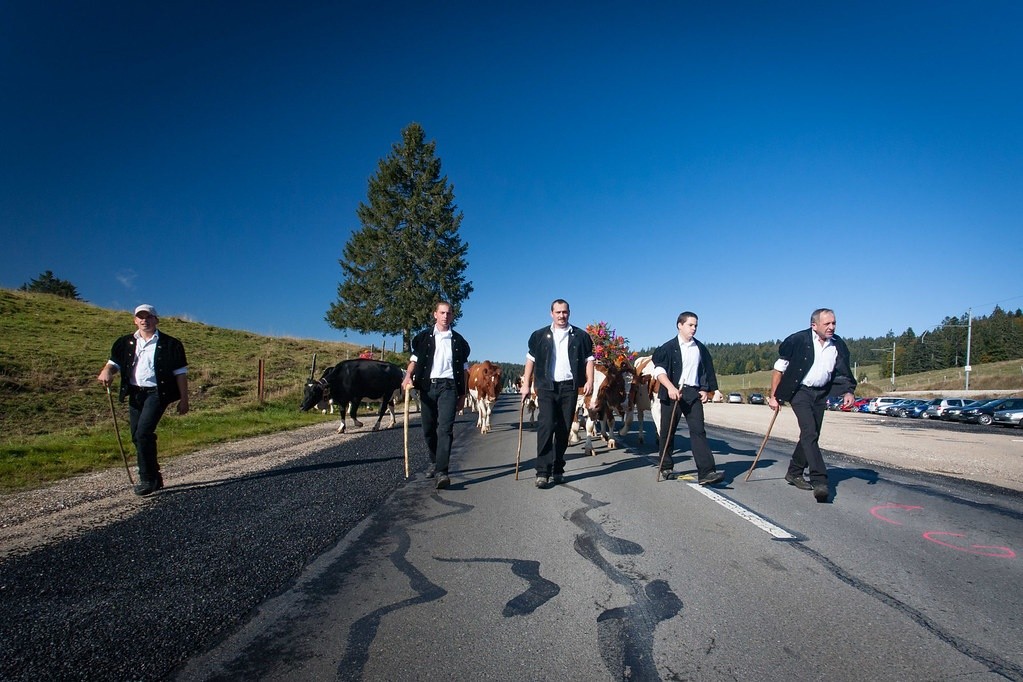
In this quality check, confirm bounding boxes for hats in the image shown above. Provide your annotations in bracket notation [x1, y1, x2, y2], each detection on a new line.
[135, 304, 158, 316]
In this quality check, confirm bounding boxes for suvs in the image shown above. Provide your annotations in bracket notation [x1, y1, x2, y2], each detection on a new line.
[925, 396, 978, 420]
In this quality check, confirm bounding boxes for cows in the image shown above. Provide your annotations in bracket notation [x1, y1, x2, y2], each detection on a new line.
[298, 357, 419, 434]
[518, 355, 661, 457]
[459, 360, 505, 435]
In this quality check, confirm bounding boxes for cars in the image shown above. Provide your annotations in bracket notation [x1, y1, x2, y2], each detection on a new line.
[726, 392, 742, 404]
[940, 398, 1000, 422]
[746, 393, 765, 405]
[958, 397, 1023, 428]
[823, 395, 932, 420]
[707, 390, 723, 402]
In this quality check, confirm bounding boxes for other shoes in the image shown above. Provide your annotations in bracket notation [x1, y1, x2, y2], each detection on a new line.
[424, 462, 435, 478]
[698, 471, 725, 487]
[535, 477, 548, 489]
[784, 471, 812, 490]
[553, 474, 565, 484]
[662, 470, 675, 481]
[134, 479, 164, 495]
[433, 472, 450, 490]
[812, 483, 830, 502]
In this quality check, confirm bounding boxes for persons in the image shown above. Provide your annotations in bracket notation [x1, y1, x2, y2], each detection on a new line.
[521, 299, 594, 488]
[401, 302, 470, 489]
[768, 308, 858, 500]
[652, 312, 724, 485]
[98, 304, 190, 496]
